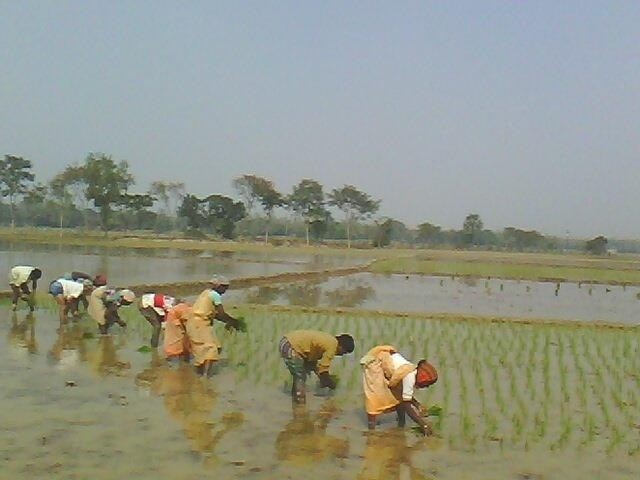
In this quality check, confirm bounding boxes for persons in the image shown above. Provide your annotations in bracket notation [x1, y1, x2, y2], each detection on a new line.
[138, 294, 185, 349]
[164, 304, 192, 362]
[185, 274, 240, 377]
[280, 331, 354, 406]
[361, 345, 438, 437]
[87, 286, 135, 336]
[49, 278, 93, 322]
[8, 265, 41, 311]
[65, 279, 93, 315]
